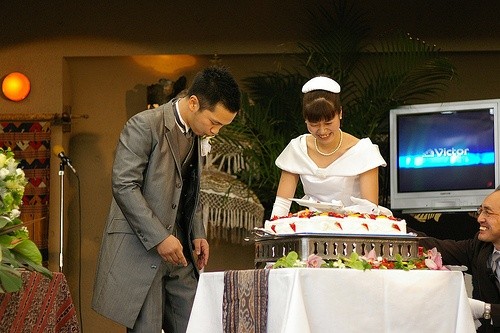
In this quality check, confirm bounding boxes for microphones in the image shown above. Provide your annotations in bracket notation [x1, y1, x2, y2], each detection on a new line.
[52, 145, 78, 177]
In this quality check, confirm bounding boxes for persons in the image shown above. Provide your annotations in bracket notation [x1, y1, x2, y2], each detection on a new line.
[407, 184, 500, 333]
[270, 76, 387, 265]
[90, 63, 241, 333]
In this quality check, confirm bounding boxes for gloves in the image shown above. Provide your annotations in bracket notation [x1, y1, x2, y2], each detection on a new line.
[341, 196, 393, 218]
[468, 298, 484, 320]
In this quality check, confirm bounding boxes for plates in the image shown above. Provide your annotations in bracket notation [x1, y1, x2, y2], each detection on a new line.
[288, 198, 342, 209]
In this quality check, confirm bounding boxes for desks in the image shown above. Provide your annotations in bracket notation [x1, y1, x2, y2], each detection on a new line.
[0, 271, 80, 333]
[186, 268, 482, 333]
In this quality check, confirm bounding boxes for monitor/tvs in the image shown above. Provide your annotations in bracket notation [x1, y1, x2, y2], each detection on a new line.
[390, 98, 500, 214]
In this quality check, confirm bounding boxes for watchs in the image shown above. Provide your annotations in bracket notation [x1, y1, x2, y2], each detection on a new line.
[484, 303, 491, 319]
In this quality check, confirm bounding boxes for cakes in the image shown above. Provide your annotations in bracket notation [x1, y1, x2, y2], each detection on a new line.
[263, 197, 407, 235]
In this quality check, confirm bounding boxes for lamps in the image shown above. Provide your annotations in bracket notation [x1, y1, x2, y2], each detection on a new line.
[0, 72, 31, 102]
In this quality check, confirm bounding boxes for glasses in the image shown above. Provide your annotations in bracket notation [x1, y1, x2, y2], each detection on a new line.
[476, 206, 500, 218]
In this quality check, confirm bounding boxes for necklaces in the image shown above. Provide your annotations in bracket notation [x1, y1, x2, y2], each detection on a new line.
[315, 128, 343, 156]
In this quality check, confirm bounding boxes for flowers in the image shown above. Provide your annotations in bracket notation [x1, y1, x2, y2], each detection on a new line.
[0, 147, 44, 293]
[268, 247, 436, 271]
